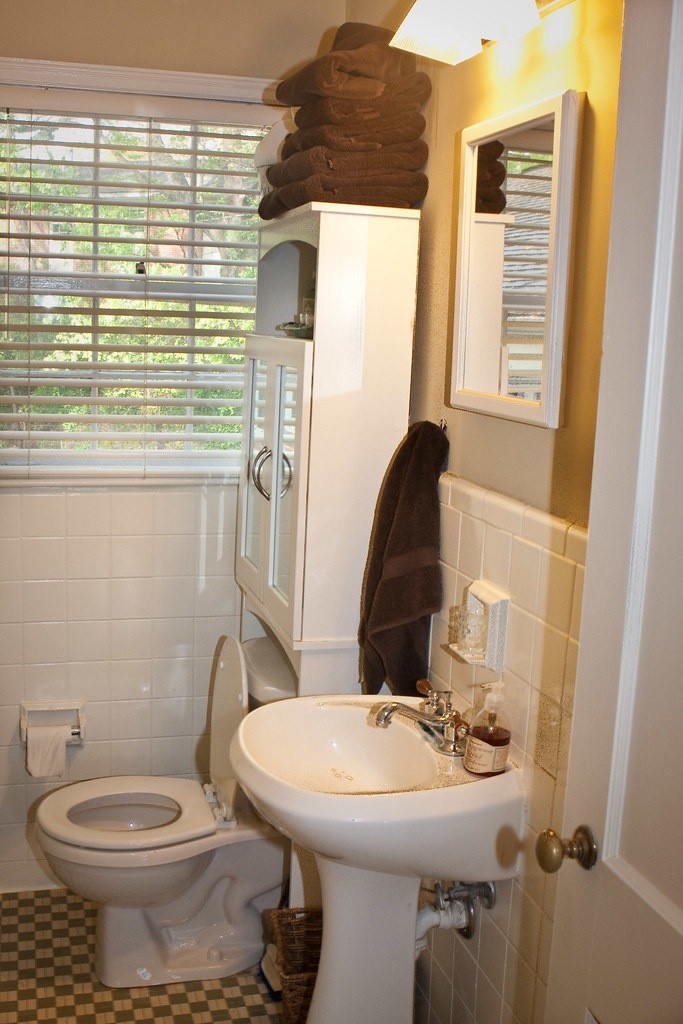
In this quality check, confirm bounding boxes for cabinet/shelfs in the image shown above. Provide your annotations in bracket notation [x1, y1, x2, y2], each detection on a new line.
[464, 213, 515, 395]
[232, 198, 422, 642]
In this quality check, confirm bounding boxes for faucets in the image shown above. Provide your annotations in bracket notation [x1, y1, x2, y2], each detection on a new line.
[367, 691, 464, 760]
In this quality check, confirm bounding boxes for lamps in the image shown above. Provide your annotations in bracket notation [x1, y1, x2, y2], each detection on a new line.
[389, 1, 541, 66]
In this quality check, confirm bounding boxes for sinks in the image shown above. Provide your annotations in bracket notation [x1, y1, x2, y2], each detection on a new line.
[228, 695, 527, 878]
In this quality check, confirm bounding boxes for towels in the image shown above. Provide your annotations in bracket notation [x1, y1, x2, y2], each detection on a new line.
[359, 420, 449, 698]
[476, 141, 507, 214]
[259, 21, 434, 220]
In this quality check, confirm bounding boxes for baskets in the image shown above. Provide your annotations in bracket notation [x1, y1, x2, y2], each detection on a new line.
[270, 906, 323, 1024]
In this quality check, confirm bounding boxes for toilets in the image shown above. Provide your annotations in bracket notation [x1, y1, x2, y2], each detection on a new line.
[34, 635, 294, 986]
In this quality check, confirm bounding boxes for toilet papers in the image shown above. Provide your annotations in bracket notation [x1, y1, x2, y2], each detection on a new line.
[26, 724, 73, 777]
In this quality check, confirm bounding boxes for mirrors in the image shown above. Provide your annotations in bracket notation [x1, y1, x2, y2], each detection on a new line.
[450, 89, 588, 431]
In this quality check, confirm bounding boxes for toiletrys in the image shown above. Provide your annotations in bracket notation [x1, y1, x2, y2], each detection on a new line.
[457, 678, 516, 777]
